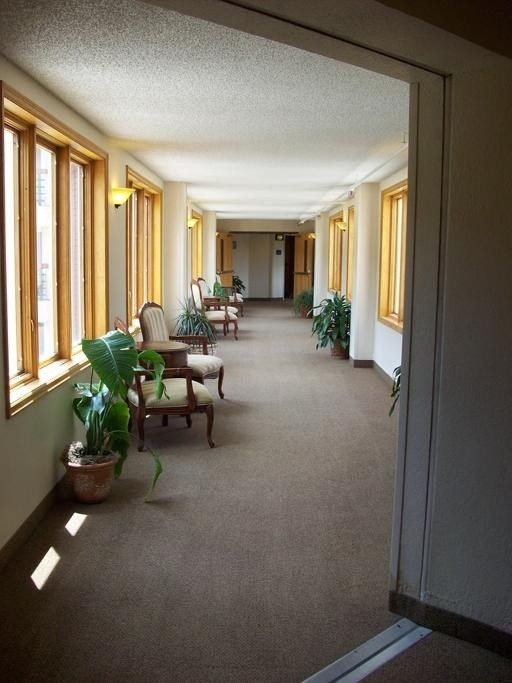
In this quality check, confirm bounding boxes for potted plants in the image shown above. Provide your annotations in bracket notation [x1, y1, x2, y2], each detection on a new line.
[286, 289, 313, 320]
[306, 291, 351, 360]
[168, 295, 218, 356]
[60, 331, 170, 505]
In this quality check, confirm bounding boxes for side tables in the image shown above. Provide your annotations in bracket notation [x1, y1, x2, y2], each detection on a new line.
[135, 341, 190, 427]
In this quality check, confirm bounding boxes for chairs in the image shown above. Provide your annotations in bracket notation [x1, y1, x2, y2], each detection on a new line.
[190, 271, 244, 341]
[138, 302, 224, 399]
[113, 318, 215, 452]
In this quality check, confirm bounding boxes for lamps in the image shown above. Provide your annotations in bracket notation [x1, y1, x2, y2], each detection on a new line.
[188, 219, 199, 230]
[335, 220, 347, 232]
[310, 232, 316, 240]
[111, 188, 136, 209]
